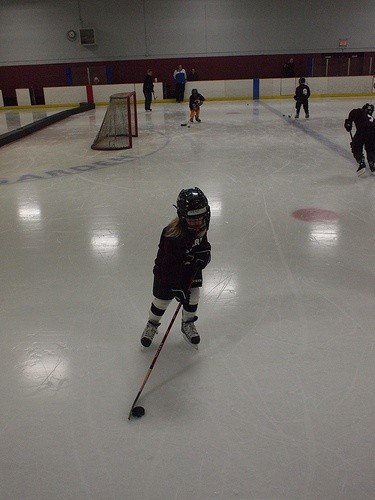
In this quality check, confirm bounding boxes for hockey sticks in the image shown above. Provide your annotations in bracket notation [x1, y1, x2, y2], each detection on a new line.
[350, 130, 368, 176]
[153, 92, 158, 100]
[181, 108, 197, 126]
[128, 294, 191, 420]
[288, 100, 296, 118]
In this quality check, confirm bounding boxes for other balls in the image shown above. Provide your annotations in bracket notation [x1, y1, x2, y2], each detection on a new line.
[247, 104, 249, 105]
[188, 126, 190, 128]
[132, 406, 145, 419]
[283, 115, 286, 116]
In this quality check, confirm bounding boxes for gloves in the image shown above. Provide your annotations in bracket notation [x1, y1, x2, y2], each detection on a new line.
[344, 119, 353, 132]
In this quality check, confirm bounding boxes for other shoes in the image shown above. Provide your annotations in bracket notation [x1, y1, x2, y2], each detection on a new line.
[196, 118, 201, 122]
[191, 120, 193, 122]
[356, 163, 367, 175]
[145, 108, 152, 111]
[371, 164, 375, 175]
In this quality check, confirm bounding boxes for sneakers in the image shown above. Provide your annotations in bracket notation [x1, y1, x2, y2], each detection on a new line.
[295, 115, 299, 118]
[141, 320, 162, 348]
[305, 114, 309, 118]
[180, 316, 200, 350]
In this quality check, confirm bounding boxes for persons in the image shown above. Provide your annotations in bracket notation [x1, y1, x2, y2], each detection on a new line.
[189, 89, 205, 123]
[140, 187, 211, 351]
[294, 78, 310, 118]
[173, 65, 187, 102]
[143, 69, 154, 111]
[284, 58, 295, 78]
[345, 103, 375, 176]
[189, 69, 199, 80]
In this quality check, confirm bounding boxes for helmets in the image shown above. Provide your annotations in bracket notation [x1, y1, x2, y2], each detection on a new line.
[299, 78, 305, 84]
[363, 103, 374, 115]
[172, 187, 210, 232]
[192, 89, 197, 96]
